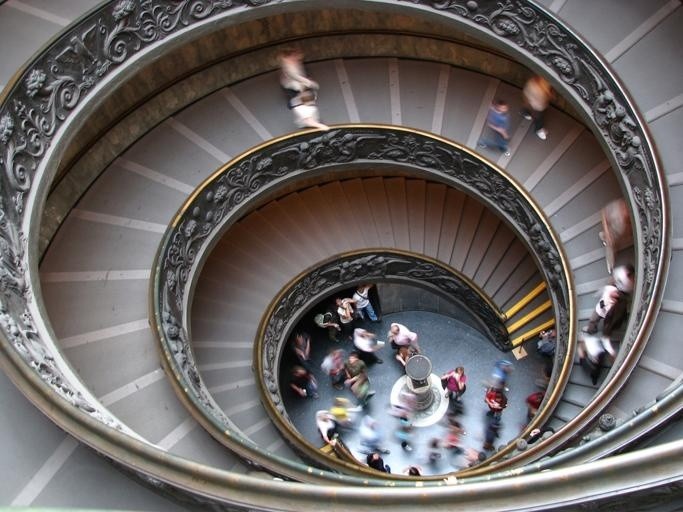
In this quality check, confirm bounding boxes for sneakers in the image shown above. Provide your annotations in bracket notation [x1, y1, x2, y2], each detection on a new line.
[536, 127, 548, 142]
[519, 110, 533, 121]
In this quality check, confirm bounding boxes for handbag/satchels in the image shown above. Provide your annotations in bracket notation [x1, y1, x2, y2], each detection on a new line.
[314, 312, 333, 329]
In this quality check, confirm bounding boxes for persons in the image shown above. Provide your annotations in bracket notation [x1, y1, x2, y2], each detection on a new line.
[289, 88, 331, 133]
[538, 328, 554, 364]
[391, 360, 517, 473]
[285, 333, 320, 400]
[315, 283, 381, 343]
[515, 388, 553, 461]
[519, 73, 557, 140]
[320, 327, 385, 411]
[387, 321, 422, 374]
[275, 47, 321, 100]
[575, 195, 644, 387]
[312, 397, 391, 474]
[475, 99, 517, 158]
